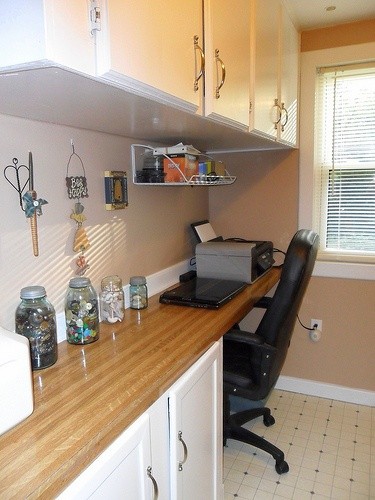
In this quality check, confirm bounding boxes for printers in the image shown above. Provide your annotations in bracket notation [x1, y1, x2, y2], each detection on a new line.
[191, 219, 276, 285]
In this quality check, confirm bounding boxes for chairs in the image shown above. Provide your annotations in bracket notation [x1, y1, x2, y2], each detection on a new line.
[220, 226, 319, 475]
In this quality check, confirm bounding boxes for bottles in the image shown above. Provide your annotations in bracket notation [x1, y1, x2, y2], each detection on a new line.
[129, 275, 148, 309]
[15, 285, 57, 371]
[99, 277, 124, 324]
[64, 276, 100, 345]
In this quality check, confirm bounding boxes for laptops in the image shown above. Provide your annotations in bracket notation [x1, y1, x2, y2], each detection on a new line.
[159, 277, 247, 310]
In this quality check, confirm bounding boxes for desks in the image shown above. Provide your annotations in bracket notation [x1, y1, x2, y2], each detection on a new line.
[0, 261, 283, 500]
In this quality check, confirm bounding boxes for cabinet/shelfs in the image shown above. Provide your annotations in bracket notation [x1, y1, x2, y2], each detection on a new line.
[55, 334, 224, 500]
[0, 0, 299, 157]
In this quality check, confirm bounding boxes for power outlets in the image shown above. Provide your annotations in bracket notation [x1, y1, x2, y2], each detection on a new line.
[309, 318, 323, 333]
